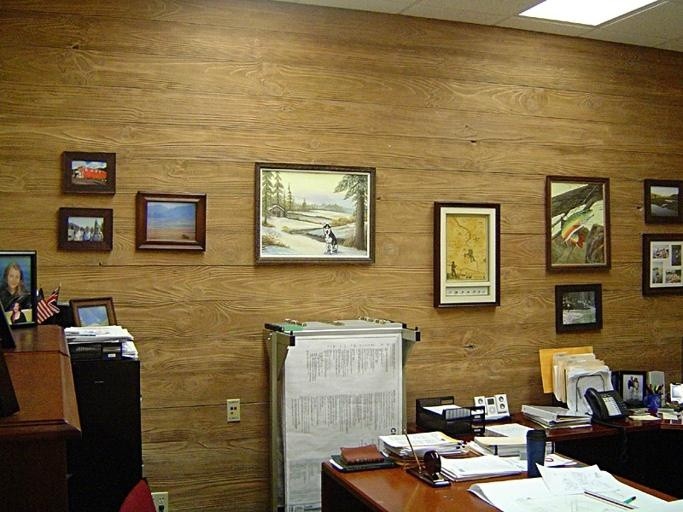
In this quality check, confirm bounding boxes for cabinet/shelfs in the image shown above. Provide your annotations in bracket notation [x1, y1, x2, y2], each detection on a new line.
[0, 324, 81, 512]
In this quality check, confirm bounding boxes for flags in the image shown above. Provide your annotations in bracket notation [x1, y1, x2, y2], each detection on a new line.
[46, 286, 60, 314]
[37, 290, 53, 324]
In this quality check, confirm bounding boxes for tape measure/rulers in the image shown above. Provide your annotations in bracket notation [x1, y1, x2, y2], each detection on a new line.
[584, 489, 640, 511]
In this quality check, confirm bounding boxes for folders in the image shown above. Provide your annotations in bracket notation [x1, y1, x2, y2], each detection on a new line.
[521, 404, 592, 423]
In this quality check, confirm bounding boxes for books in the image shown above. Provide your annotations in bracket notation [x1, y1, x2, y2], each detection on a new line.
[329, 444, 395, 472]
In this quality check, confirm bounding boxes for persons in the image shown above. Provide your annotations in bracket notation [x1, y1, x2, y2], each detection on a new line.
[11, 302, 26, 324]
[0, 262, 31, 313]
[69, 226, 104, 242]
[628, 376, 639, 400]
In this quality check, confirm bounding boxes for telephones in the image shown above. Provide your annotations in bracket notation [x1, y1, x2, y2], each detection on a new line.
[584, 388, 629, 419]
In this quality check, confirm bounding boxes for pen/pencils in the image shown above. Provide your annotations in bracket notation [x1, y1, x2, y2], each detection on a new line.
[470, 449, 481, 456]
[624, 496, 636, 503]
[545, 458, 553, 462]
[644, 384, 663, 394]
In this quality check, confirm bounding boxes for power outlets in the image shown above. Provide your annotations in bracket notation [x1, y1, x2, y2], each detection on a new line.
[150, 492, 169, 512]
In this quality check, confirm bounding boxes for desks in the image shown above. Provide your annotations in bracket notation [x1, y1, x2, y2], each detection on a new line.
[405, 400, 682, 475]
[321, 431, 682, 512]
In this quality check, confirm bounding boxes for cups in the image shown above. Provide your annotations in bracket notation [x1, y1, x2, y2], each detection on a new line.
[643, 393, 661, 408]
[525, 430, 546, 477]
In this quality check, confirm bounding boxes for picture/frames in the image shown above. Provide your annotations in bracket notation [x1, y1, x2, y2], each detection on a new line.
[69, 298, 116, 326]
[642, 233, 683, 296]
[253, 162, 376, 266]
[135, 192, 209, 252]
[644, 179, 683, 225]
[63, 152, 116, 196]
[59, 207, 113, 252]
[434, 201, 500, 308]
[555, 283, 603, 334]
[0, 252, 37, 328]
[545, 175, 611, 272]
